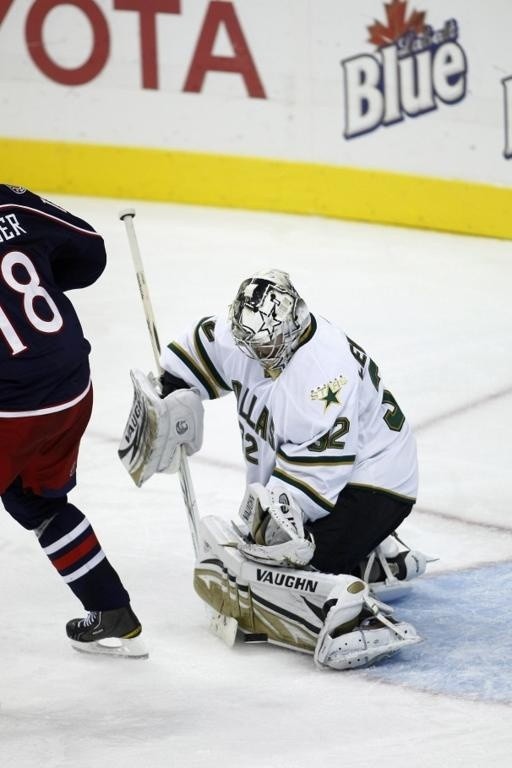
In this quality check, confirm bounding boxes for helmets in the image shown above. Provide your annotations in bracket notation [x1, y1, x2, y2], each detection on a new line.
[228, 271, 311, 370]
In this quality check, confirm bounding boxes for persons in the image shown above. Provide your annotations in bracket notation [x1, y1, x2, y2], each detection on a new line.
[124, 263, 431, 670]
[2, 183, 144, 641]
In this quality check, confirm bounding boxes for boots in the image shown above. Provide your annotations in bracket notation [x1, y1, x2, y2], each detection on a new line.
[376, 552, 426, 582]
[63, 608, 149, 657]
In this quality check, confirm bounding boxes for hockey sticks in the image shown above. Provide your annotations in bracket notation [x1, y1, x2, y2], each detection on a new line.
[120, 208, 238, 648]
